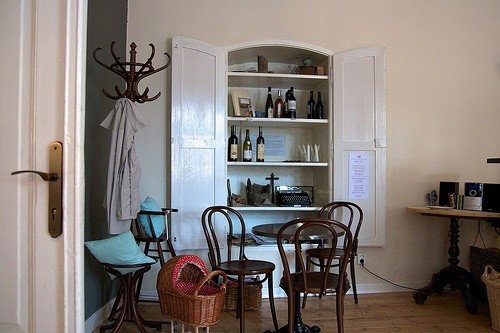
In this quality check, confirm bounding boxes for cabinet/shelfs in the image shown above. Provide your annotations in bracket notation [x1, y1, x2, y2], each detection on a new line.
[171, 33, 387, 251]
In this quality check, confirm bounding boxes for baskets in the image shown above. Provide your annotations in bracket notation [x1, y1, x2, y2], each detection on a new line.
[220, 283, 262, 311]
[481, 265, 500, 333]
[156, 255, 226, 327]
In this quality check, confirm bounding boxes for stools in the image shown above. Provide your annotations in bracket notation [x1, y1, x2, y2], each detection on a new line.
[107, 206, 179, 324]
[98, 255, 163, 333]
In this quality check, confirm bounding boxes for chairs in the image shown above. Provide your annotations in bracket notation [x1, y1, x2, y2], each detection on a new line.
[300, 201, 363, 310]
[276, 217, 353, 333]
[201, 205, 279, 333]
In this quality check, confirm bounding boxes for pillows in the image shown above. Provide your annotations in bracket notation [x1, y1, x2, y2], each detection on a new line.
[137, 195, 166, 239]
[84, 230, 156, 280]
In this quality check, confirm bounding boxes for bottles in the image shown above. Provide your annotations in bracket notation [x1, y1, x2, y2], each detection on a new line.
[265, 86, 274, 118]
[243, 129, 253, 162]
[284, 87, 297, 119]
[315, 91, 324, 119]
[274, 90, 284, 118]
[307, 91, 315, 119]
[256, 126, 265, 162]
[228, 124, 238, 161]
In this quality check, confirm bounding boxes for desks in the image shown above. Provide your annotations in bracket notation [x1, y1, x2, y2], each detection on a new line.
[251, 222, 345, 333]
[406, 203, 500, 316]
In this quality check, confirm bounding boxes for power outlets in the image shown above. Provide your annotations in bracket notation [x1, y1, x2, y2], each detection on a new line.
[357, 252, 366, 265]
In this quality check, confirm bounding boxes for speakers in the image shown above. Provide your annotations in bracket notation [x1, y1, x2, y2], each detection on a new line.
[439, 182, 459, 207]
[482, 183, 500, 213]
